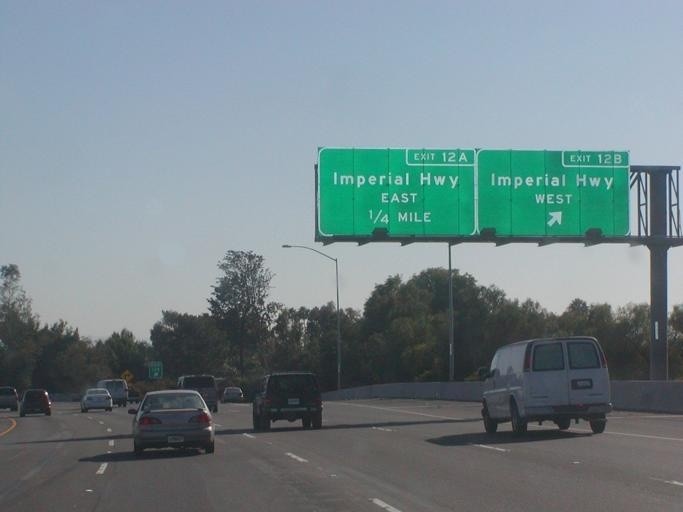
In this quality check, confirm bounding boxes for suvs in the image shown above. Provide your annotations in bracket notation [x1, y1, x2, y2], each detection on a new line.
[252, 371, 323, 430]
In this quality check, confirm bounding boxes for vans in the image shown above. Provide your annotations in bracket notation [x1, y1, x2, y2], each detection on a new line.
[97, 378, 129, 408]
[177, 374, 220, 414]
[481, 335, 612, 435]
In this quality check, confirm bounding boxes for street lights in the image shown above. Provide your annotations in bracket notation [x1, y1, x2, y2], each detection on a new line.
[282, 245, 341, 390]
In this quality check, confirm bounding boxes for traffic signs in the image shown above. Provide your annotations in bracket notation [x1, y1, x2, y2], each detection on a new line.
[317, 149, 630, 242]
[147, 360, 164, 380]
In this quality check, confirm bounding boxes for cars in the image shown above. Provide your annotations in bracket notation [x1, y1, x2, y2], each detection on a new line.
[20, 388, 52, 418]
[81, 387, 113, 413]
[1, 385, 21, 411]
[221, 386, 244, 404]
[128, 388, 215, 458]
